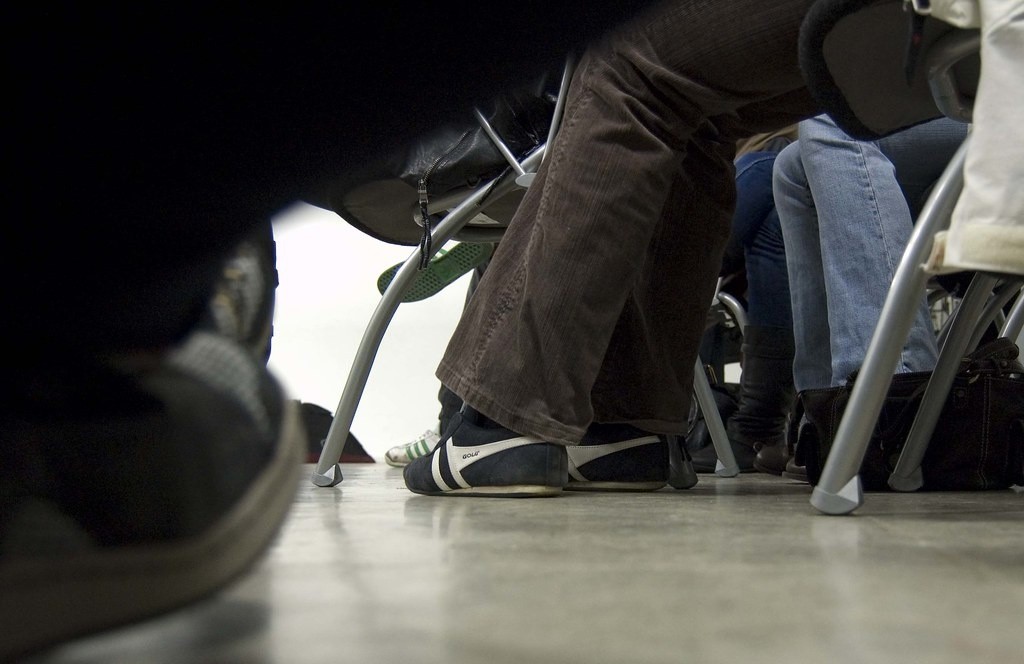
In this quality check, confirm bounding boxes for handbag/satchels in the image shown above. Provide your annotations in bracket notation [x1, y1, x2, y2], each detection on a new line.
[786, 337, 1024, 492]
[396, 89, 549, 270]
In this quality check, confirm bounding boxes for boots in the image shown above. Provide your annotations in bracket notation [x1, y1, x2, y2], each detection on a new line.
[691, 325, 793, 471]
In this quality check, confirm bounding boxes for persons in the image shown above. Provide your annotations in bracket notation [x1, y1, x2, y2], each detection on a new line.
[403, 0, 1024, 497]
[772, 112, 971, 482]
[0, 0, 659, 660]
[684, 124, 799, 475]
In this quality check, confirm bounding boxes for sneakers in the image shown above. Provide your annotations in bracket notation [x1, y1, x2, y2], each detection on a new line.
[404, 412, 568, 494]
[384, 429, 442, 467]
[376, 239, 495, 303]
[567, 430, 669, 489]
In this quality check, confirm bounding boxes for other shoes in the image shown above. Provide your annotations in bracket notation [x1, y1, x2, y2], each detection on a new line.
[753, 443, 789, 474]
[0, 329, 304, 651]
[783, 457, 808, 481]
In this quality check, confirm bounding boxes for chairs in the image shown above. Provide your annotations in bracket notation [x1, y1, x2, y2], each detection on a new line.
[310, 51, 1022, 518]
